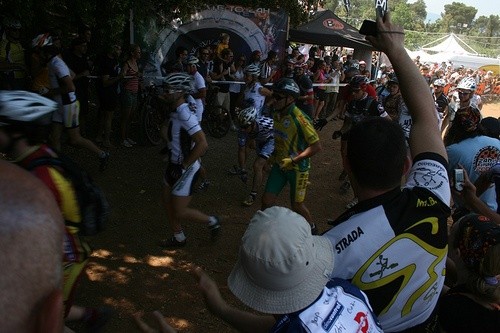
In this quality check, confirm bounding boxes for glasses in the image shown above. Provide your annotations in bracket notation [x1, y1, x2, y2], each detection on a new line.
[201, 52, 210, 56]
[227, 55, 235, 59]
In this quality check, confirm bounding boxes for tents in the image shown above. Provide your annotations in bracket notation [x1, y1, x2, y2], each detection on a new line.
[290, 9, 383, 52]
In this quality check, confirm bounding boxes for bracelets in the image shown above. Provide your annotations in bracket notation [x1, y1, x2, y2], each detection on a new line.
[291, 156, 297, 165]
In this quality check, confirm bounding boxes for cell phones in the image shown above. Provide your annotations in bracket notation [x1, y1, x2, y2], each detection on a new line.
[454, 169, 465, 191]
[359, 20, 377, 37]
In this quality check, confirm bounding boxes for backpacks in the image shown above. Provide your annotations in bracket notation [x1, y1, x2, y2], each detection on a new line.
[32, 143, 110, 236]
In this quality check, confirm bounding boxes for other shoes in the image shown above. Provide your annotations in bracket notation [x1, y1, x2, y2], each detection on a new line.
[243, 184, 268, 205]
[80, 303, 115, 333]
[228, 164, 251, 182]
[336, 169, 360, 209]
[98, 150, 114, 172]
[207, 213, 222, 236]
[193, 182, 210, 192]
[160, 234, 188, 247]
[311, 221, 318, 235]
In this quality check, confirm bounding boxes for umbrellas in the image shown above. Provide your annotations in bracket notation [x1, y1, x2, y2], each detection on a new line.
[422, 32, 479, 56]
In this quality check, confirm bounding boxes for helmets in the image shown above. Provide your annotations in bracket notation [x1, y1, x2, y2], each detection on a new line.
[32, 33, 53, 48]
[236, 106, 258, 128]
[388, 77, 400, 85]
[164, 72, 196, 95]
[0, 90, 59, 123]
[185, 56, 199, 65]
[433, 78, 447, 88]
[243, 64, 262, 77]
[7, 19, 22, 29]
[270, 77, 301, 97]
[348, 74, 371, 90]
[454, 77, 477, 94]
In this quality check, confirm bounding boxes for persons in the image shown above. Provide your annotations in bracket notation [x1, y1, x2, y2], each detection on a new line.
[316, 6, 451, 333]
[0, 0, 500, 333]
[187, 205, 387, 333]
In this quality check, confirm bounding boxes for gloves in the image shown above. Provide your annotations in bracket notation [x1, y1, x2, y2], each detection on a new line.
[313, 117, 328, 131]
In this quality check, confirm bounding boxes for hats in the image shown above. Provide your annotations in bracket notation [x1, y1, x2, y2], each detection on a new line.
[227, 205, 336, 315]
